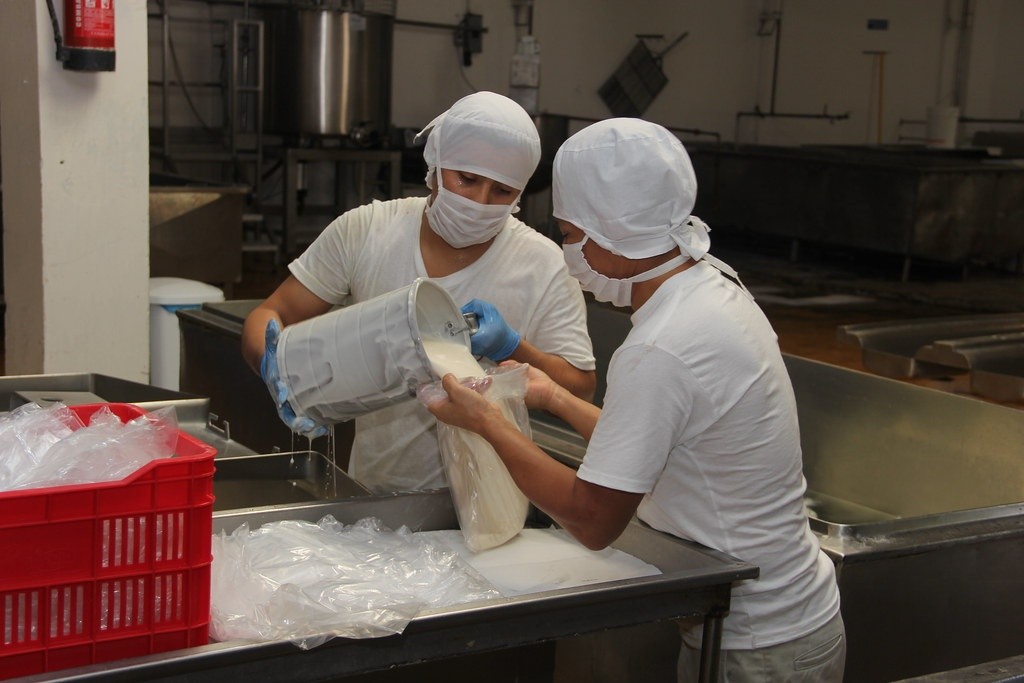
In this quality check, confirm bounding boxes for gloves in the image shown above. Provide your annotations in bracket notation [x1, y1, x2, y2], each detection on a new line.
[260, 319, 330, 439]
[460, 299, 520, 361]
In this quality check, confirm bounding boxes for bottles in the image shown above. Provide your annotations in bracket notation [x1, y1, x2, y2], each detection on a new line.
[506, 37, 540, 115]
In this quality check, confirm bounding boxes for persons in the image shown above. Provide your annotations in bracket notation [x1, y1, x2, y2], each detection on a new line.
[418, 117, 847, 683]
[244, 92, 596, 496]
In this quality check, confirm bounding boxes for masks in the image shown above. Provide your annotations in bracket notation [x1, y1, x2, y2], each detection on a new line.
[426, 167, 522, 249]
[562, 216, 692, 306]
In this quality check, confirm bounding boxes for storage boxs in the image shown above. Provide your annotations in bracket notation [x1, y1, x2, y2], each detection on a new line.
[0, 402, 217, 681]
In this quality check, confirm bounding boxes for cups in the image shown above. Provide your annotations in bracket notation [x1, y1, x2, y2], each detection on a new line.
[926, 105, 960, 148]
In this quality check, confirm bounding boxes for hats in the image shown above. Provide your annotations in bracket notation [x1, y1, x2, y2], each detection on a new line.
[424, 91, 542, 192]
[553, 116, 697, 259]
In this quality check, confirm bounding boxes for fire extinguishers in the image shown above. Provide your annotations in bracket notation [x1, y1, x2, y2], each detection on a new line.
[45, 1, 117, 73]
